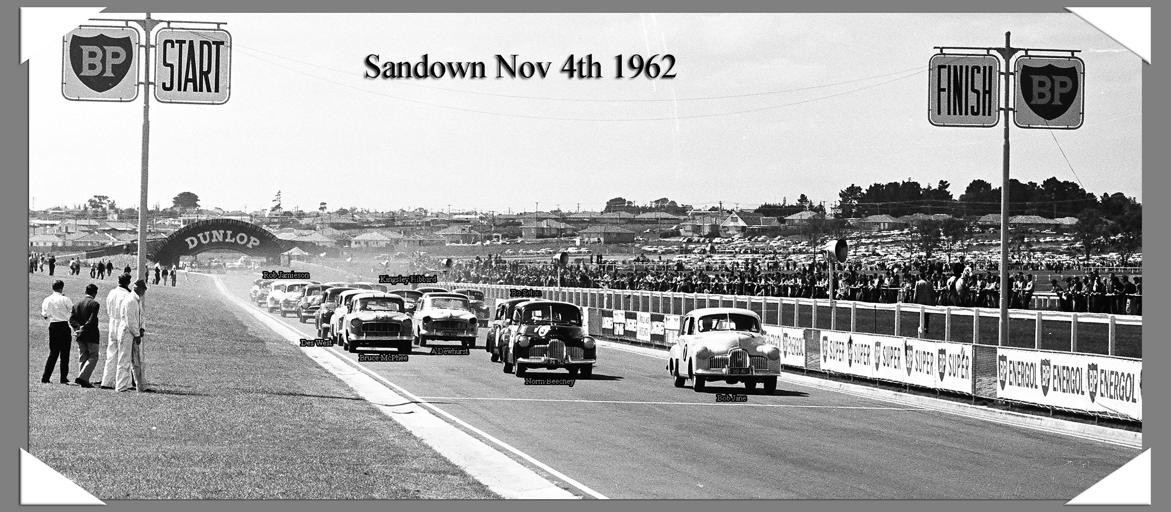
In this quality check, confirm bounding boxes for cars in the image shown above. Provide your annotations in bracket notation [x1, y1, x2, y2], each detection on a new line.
[187, 251, 258, 271]
[250, 276, 490, 354]
[486, 297, 600, 379]
[662, 305, 783, 393]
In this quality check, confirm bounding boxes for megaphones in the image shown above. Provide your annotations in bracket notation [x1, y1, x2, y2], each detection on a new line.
[553, 252, 569, 266]
[820, 239, 848, 263]
[441, 259, 453, 267]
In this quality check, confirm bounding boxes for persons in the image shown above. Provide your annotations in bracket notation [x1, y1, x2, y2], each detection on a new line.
[40, 279, 75, 384]
[29, 251, 178, 287]
[100, 272, 132, 390]
[408, 251, 1144, 309]
[69, 283, 102, 388]
[113, 281, 158, 394]
